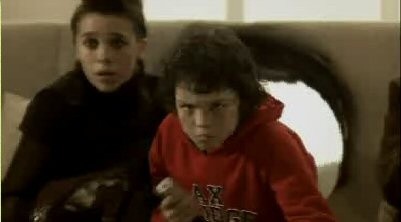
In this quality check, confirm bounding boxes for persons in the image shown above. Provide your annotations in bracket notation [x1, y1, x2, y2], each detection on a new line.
[150, 28, 339, 221]
[375, 78, 401, 222]
[2, 1, 172, 222]
